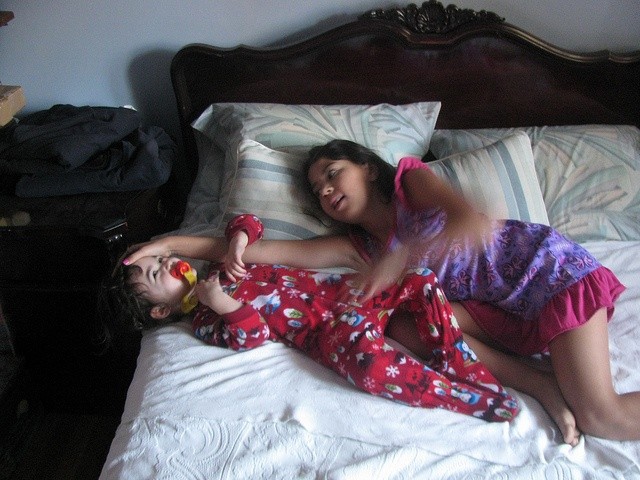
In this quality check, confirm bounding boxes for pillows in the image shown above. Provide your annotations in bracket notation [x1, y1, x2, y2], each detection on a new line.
[426, 124, 640, 246]
[188, 99, 442, 220]
[232, 131, 556, 246]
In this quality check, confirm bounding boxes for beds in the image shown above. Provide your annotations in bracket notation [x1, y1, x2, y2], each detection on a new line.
[87, 0, 640, 480]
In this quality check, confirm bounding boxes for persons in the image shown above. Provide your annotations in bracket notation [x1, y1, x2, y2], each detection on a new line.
[120, 138, 640, 450]
[89, 212, 523, 425]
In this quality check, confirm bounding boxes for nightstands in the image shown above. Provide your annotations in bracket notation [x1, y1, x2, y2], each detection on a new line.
[0, 189, 177, 416]
